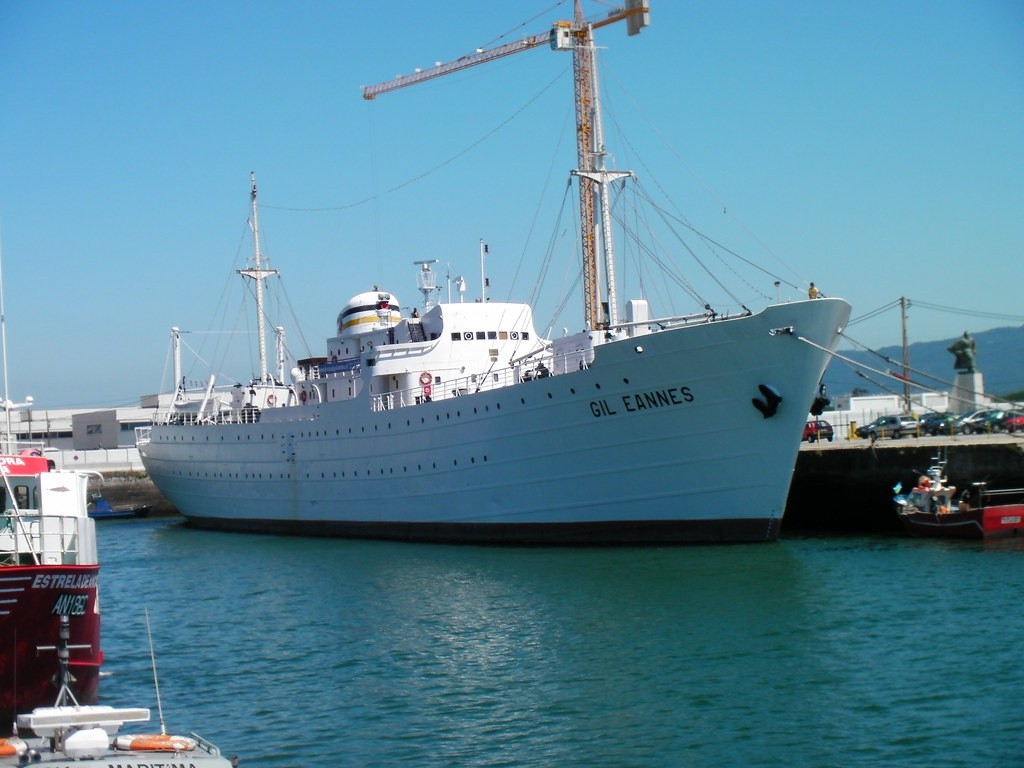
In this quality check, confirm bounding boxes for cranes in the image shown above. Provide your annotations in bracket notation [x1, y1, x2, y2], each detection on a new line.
[358, 0, 652, 338]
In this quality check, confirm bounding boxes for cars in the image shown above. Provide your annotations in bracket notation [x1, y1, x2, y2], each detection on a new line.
[854, 414, 920, 440]
[801, 420, 834, 444]
[918, 409, 1024, 436]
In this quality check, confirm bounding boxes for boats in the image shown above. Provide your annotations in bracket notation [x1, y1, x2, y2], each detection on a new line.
[87, 492, 154, 520]
[890, 449, 1024, 545]
[1, 605, 239, 768]
[0, 274, 105, 729]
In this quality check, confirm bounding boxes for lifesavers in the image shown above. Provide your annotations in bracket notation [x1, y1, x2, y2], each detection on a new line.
[0, 738, 30, 758]
[268, 395, 277, 404]
[113, 734, 195, 751]
[420, 372, 433, 385]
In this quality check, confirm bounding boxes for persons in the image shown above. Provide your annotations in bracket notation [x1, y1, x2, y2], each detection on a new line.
[948, 331, 976, 373]
[959, 500, 969, 510]
[536, 363, 548, 377]
[412, 308, 419, 318]
[930, 496, 941, 512]
[808, 283, 826, 299]
[17, 494, 24, 508]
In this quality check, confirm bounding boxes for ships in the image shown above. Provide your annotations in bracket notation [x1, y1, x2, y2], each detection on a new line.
[129, 20, 854, 548]
